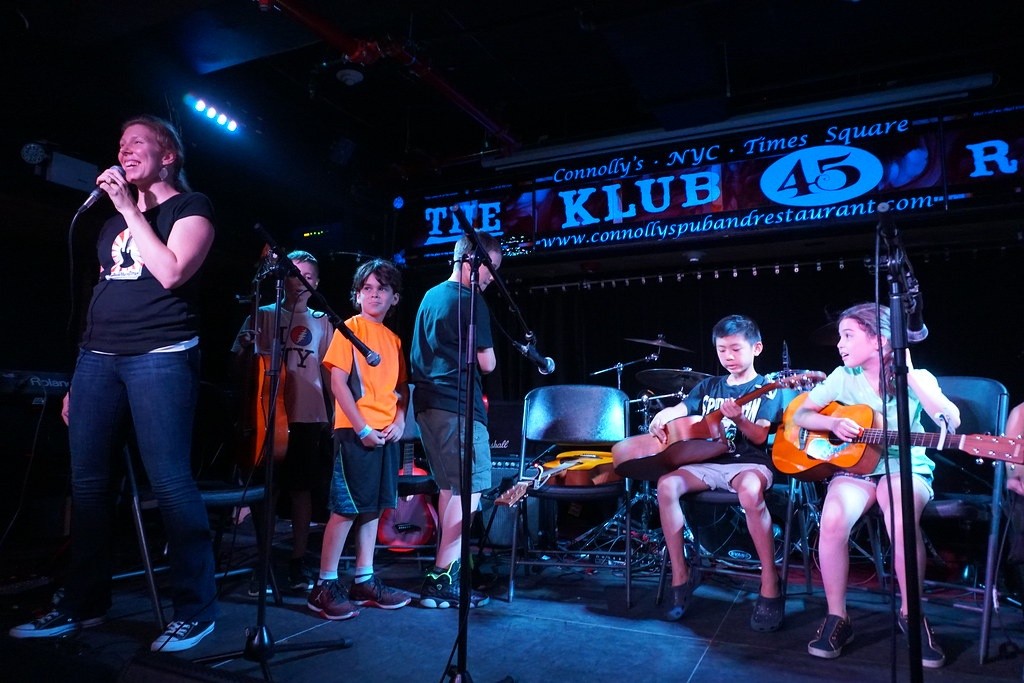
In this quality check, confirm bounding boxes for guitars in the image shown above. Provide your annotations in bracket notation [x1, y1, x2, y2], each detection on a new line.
[377, 441, 440, 554]
[493, 449, 623, 509]
[611, 369, 827, 482]
[772, 391, 1024, 481]
[233, 248, 289, 468]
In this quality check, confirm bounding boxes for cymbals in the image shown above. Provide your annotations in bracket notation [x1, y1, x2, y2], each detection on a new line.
[808, 321, 840, 346]
[623, 337, 691, 351]
[635, 368, 715, 395]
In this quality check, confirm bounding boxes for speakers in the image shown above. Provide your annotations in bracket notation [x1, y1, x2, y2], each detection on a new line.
[680, 484, 800, 565]
[477, 454, 554, 550]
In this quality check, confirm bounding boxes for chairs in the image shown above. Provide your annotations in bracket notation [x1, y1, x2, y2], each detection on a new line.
[344, 384, 442, 571]
[112, 380, 283, 634]
[508, 384, 633, 608]
[655, 388, 812, 614]
[847, 376, 1009, 665]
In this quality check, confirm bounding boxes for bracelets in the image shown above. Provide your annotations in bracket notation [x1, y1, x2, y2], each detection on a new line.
[357, 424, 372, 440]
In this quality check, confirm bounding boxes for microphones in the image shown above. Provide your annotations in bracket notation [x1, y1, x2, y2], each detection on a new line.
[782, 341, 791, 366]
[906, 280, 930, 342]
[77, 165, 126, 214]
[481, 495, 496, 501]
[773, 523, 782, 540]
[396, 524, 421, 532]
[512, 342, 556, 375]
[646, 390, 664, 411]
[328, 317, 381, 367]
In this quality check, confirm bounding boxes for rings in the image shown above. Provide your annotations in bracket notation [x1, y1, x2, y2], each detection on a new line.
[106, 178, 114, 183]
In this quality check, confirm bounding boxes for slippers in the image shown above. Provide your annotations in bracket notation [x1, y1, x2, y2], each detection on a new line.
[751, 572, 787, 633]
[663, 566, 702, 621]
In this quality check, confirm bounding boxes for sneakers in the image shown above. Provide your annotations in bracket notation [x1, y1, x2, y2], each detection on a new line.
[151, 620, 216, 652]
[9, 606, 107, 638]
[347, 573, 412, 610]
[304, 580, 360, 621]
[470, 553, 498, 591]
[420, 560, 490, 610]
[806, 614, 854, 658]
[898, 608, 945, 668]
[247, 568, 275, 597]
[287, 557, 311, 590]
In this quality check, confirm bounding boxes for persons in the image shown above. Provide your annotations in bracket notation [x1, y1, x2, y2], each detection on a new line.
[9, 114, 216, 653]
[648, 314, 785, 631]
[230, 250, 335, 597]
[409, 231, 502, 609]
[306, 259, 411, 621]
[793, 302, 961, 668]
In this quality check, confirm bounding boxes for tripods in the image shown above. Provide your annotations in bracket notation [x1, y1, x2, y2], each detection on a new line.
[565, 346, 689, 550]
[187, 223, 352, 683]
